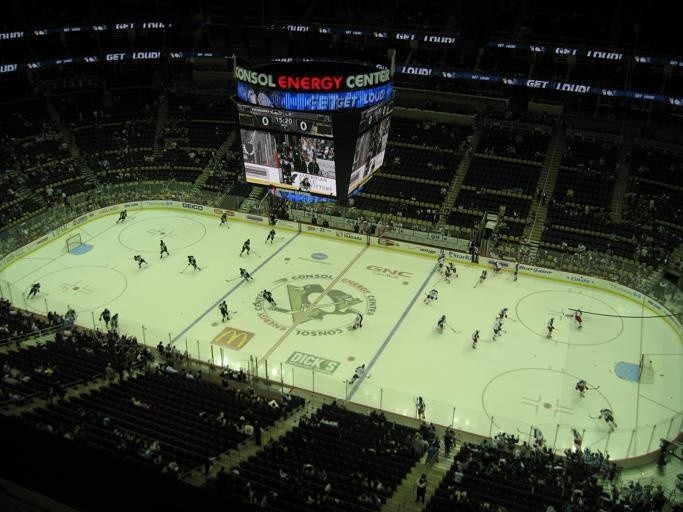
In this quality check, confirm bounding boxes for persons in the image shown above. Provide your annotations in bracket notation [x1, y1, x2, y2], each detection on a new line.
[1, 81, 681, 306]
[1, 281, 683, 512]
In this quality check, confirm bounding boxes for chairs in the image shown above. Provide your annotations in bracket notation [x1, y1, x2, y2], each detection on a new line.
[1, 86, 683, 512]
